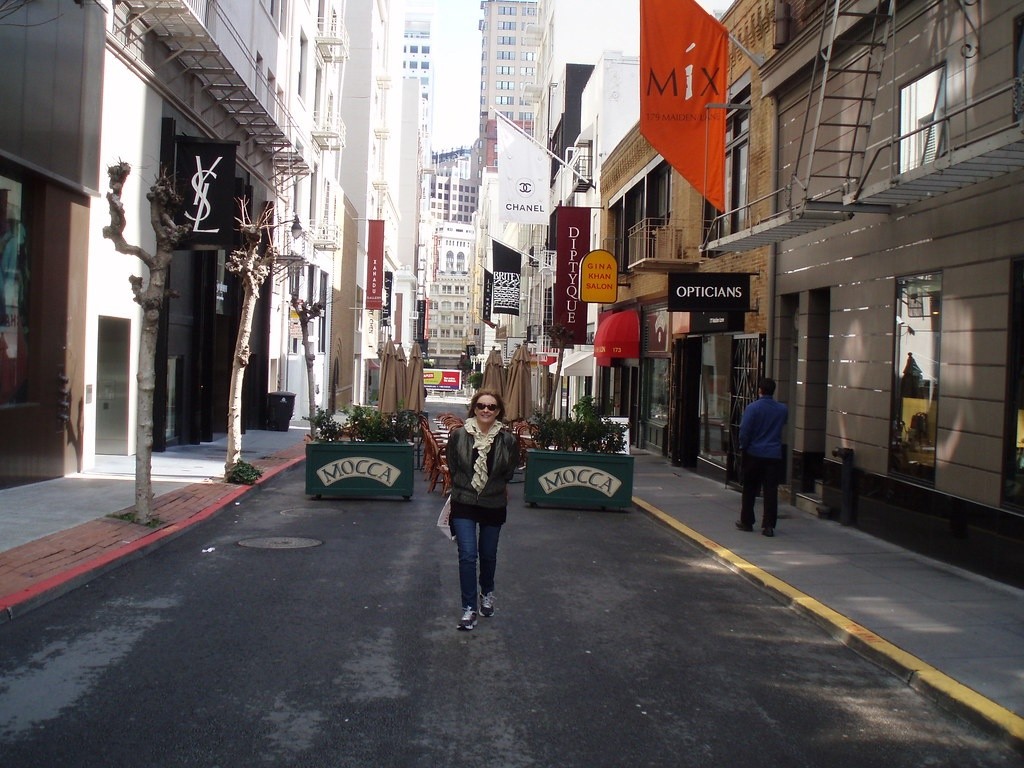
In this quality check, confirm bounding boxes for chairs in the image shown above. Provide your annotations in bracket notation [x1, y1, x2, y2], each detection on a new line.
[304, 413, 578, 496]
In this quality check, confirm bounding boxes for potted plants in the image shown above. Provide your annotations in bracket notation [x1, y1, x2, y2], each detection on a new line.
[524, 393, 635, 512]
[301, 399, 426, 501]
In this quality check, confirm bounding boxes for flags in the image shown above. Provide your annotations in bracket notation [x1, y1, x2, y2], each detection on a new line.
[477, 267, 498, 329]
[497, 117, 552, 224]
[492, 239, 521, 317]
[637, 1, 728, 212]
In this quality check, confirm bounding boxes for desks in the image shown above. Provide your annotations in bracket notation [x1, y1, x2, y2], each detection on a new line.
[519, 434, 537, 448]
[439, 426, 448, 429]
[433, 421, 444, 424]
[440, 433, 450, 439]
[544, 446, 559, 451]
[435, 430, 449, 434]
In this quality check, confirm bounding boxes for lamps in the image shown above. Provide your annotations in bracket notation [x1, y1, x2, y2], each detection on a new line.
[272, 214, 302, 240]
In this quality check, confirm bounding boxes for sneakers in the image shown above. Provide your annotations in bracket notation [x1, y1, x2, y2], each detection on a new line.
[456, 607, 477, 630]
[479, 592, 496, 617]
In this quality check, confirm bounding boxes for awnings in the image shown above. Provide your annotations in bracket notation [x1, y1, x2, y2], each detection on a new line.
[594, 309, 640, 359]
[549, 351, 593, 376]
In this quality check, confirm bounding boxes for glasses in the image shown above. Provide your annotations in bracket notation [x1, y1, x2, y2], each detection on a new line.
[476, 403, 498, 411]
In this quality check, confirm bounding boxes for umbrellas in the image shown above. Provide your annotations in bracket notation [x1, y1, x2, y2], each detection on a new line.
[482, 345, 505, 400]
[506, 344, 521, 387]
[503, 340, 532, 474]
[378, 334, 407, 416]
[406, 337, 424, 470]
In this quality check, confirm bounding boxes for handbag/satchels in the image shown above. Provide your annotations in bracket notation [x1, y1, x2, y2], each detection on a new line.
[437, 495, 456, 541]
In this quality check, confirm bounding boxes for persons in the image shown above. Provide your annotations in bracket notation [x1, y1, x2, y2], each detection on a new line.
[735, 378, 789, 537]
[445, 389, 518, 630]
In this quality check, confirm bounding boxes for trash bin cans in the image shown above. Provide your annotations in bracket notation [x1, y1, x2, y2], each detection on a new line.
[267, 392, 297, 432]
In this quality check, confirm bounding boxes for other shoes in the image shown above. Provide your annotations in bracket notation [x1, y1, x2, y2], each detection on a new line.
[736, 520, 753, 532]
[762, 528, 773, 536]
